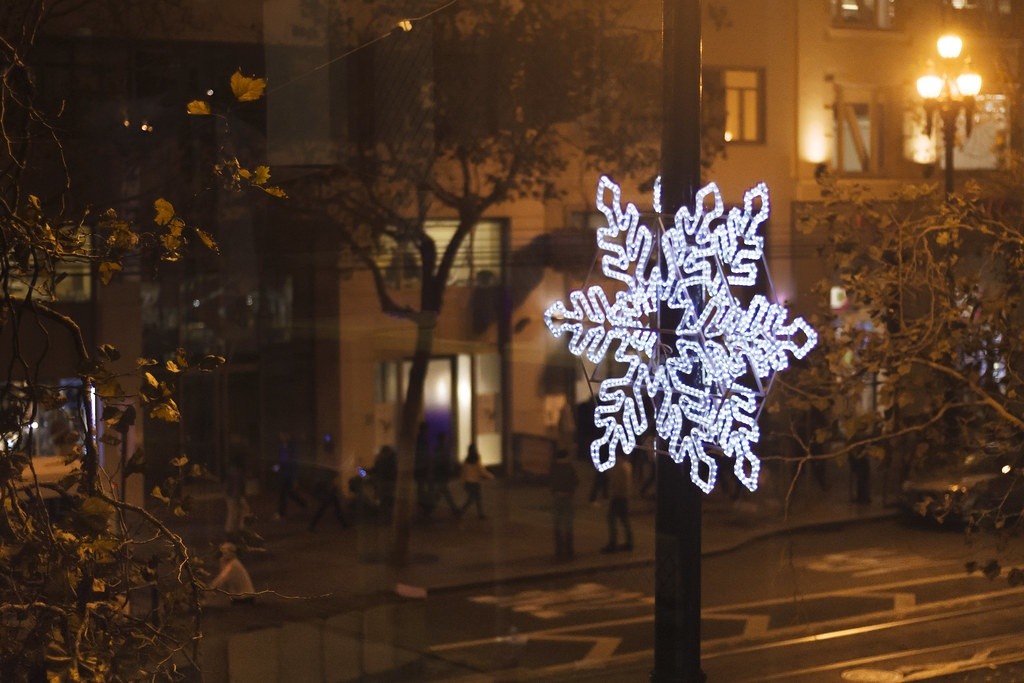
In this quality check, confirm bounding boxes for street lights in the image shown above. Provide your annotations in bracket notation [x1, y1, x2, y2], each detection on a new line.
[911, 25, 984, 451]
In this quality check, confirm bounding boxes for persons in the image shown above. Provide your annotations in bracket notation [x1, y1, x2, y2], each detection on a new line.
[368, 447, 464, 521]
[307, 463, 348, 530]
[600, 452, 634, 553]
[273, 429, 309, 518]
[206, 543, 258, 630]
[454, 443, 495, 521]
[226, 453, 266, 556]
[546, 449, 583, 564]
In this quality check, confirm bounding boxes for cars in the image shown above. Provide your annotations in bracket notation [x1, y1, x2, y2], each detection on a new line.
[897, 440, 1024, 531]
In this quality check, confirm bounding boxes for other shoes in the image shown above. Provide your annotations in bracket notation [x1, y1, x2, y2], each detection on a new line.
[599, 542, 619, 552]
[619, 543, 633, 549]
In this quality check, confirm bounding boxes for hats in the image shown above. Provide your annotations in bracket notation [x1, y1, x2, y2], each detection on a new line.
[219, 542, 235, 552]
